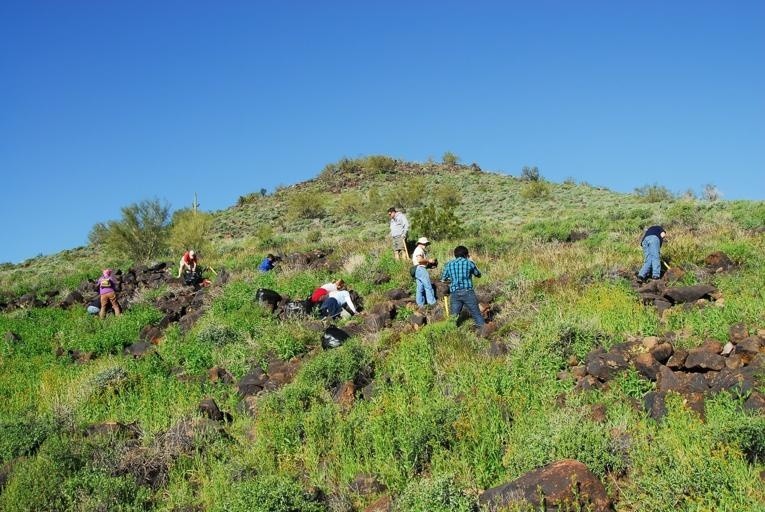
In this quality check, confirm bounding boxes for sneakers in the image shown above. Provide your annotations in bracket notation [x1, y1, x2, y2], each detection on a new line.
[636, 276, 644, 282]
[652, 276, 660, 281]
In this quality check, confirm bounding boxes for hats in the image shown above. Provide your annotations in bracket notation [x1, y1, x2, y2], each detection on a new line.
[189, 250, 194, 259]
[415, 237, 431, 246]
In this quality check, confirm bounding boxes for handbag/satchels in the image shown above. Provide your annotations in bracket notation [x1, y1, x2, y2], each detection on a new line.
[101, 278, 111, 287]
[410, 265, 416, 277]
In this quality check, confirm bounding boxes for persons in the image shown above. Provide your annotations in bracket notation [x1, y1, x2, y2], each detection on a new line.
[387, 207, 409, 262]
[176, 250, 197, 278]
[311, 278, 345, 304]
[258, 253, 276, 272]
[96, 269, 120, 320]
[412, 237, 437, 306]
[637, 225, 666, 283]
[440, 245, 485, 328]
[319, 289, 359, 323]
